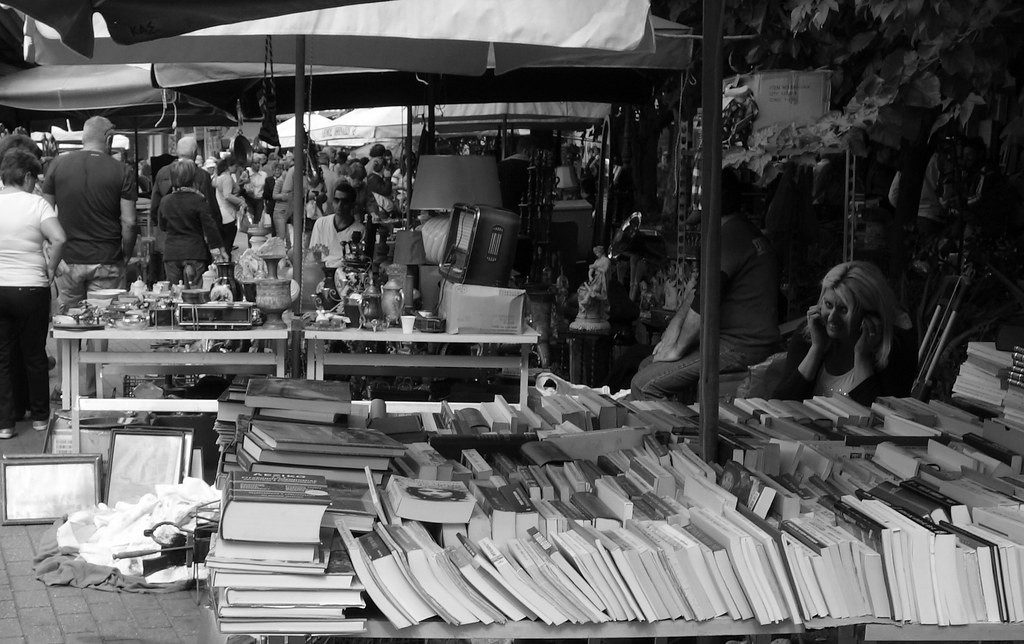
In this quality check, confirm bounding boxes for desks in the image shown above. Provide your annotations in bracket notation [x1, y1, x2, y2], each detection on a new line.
[49, 321, 288, 454]
[300, 326, 541, 411]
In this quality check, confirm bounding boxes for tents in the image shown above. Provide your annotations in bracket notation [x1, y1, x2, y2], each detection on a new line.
[0, 0, 694, 380]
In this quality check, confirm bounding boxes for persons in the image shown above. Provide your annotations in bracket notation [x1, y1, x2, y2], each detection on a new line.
[0, 115, 1005, 439]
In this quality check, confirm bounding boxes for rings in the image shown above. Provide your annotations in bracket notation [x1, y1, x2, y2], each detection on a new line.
[869, 331, 876, 339]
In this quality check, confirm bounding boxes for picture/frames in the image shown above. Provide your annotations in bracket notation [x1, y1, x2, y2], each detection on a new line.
[0, 425, 195, 525]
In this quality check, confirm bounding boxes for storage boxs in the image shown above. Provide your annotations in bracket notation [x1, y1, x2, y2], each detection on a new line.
[420, 263, 445, 312]
[437, 278, 528, 335]
[722, 70, 833, 135]
[551, 198, 593, 254]
[51, 374, 222, 481]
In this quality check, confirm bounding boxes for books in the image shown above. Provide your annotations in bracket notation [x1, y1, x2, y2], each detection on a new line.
[214, 343, 1023, 635]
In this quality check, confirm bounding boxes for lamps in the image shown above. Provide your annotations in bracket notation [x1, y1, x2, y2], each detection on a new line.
[555, 165, 578, 201]
[393, 230, 426, 317]
[410, 153, 502, 211]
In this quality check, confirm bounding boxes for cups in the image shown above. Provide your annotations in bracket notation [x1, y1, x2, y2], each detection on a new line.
[400, 315, 416, 334]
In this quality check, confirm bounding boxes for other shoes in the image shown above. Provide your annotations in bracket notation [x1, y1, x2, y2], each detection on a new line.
[0, 427, 17, 438]
[31, 418, 49, 430]
